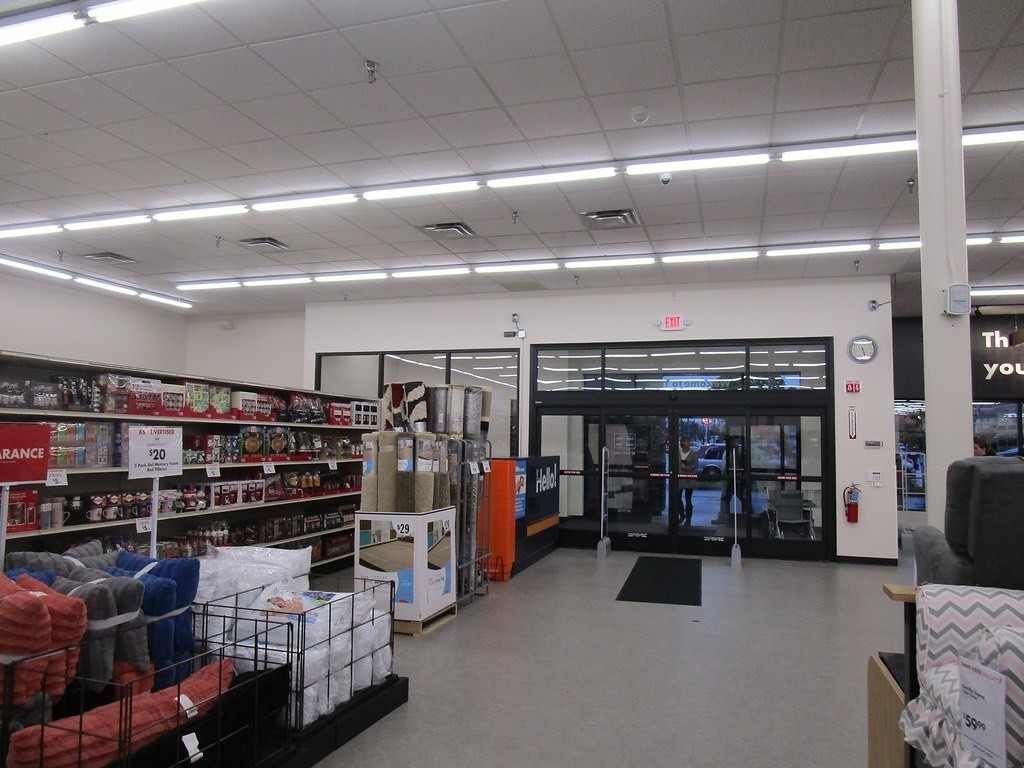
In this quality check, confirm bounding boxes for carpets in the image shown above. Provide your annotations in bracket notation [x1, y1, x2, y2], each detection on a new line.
[615, 556, 702, 607]
[662, 527, 717, 535]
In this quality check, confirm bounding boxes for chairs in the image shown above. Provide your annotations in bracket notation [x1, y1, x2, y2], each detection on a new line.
[762, 489, 816, 540]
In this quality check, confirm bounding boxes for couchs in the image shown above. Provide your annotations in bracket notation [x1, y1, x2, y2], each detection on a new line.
[913, 456, 1024, 587]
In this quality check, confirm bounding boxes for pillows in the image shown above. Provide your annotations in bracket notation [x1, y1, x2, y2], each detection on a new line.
[5, 539, 103, 571]
[54, 547, 125, 579]
[0, 572, 55, 705]
[105, 567, 177, 662]
[68, 566, 150, 674]
[16, 574, 88, 703]
[49, 575, 118, 693]
[116, 551, 200, 652]
[5, 656, 234, 768]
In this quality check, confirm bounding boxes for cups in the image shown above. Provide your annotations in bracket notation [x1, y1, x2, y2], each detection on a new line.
[85, 501, 174, 520]
[51, 511, 70, 526]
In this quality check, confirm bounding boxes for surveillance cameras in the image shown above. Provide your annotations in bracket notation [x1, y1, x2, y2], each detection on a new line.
[660, 171, 672, 186]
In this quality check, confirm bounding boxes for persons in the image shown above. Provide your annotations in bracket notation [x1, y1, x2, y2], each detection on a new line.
[711, 434, 755, 526]
[677, 435, 698, 528]
[264, 595, 305, 618]
[974, 434, 998, 456]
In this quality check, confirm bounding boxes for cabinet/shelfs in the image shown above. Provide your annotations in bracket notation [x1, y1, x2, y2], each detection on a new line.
[0, 350, 383, 579]
[867, 584, 932, 768]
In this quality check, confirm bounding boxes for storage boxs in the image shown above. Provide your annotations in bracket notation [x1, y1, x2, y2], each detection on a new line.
[211, 406, 238, 419]
[303, 514, 323, 535]
[323, 402, 352, 426]
[160, 405, 187, 417]
[161, 383, 186, 411]
[370, 403, 378, 427]
[171, 434, 266, 509]
[266, 426, 289, 457]
[185, 405, 212, 418]
[362, 402, 370, 427]
[351, 401, 362, 426]
[0, 489, 40, 534]
[230, 391, 258, 420]
[323, 511, 344, 531]
[129, 377, 161, 408]
[228, 515, 304, 546]
[29, 380, 63, 411]
[266, 454, 290, 462]
[127, 397, 160, 416]
[238, 425, 266, 458]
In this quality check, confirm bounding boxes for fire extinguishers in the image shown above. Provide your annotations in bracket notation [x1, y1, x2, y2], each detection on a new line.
[848, 482, 862, 523]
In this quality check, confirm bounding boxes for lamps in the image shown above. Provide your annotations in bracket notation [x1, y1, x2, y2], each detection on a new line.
[1008, 299, 1024, 348]
[0, 0, 1024, 310]
[974, 306, 983, 318]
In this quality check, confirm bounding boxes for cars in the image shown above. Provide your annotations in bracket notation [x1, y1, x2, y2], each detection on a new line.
[666, 440, 782, 482]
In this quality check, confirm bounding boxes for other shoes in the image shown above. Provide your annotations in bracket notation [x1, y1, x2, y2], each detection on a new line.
[711, 513, 730, 525]
[678, 513, 686, 523]
[684, 517, 691, 526]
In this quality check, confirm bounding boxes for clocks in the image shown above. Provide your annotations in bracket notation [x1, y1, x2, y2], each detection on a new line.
[846, 335, 878, 364]
